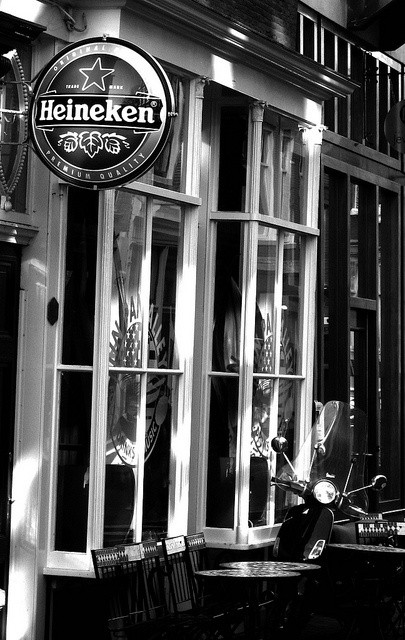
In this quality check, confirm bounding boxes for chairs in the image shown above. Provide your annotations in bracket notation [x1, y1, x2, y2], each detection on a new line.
[86, 544, 208, 639]
[137, 540, 219, 631]
[185, 531, 215, 605]
[160, 535, 243, 626]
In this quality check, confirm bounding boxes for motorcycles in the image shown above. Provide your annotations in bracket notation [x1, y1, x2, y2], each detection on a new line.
[243, 400, 405, 640]
